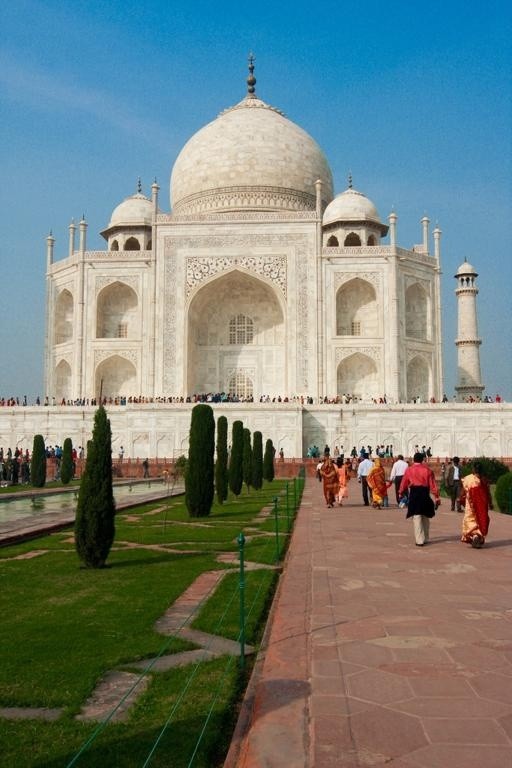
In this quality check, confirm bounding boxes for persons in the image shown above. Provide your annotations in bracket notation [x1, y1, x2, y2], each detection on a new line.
[142, 458, 150, 478]
[1, 393, 502, 407]
[0, 444, 125, 486]
[279, 447, 285, 463]
[307, 443, 493, 549]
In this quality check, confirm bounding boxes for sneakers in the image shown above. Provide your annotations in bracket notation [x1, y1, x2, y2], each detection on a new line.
[328, 502, 342, 507]
[472, 537, 479, 548]
[451, 507, 463, 512]
